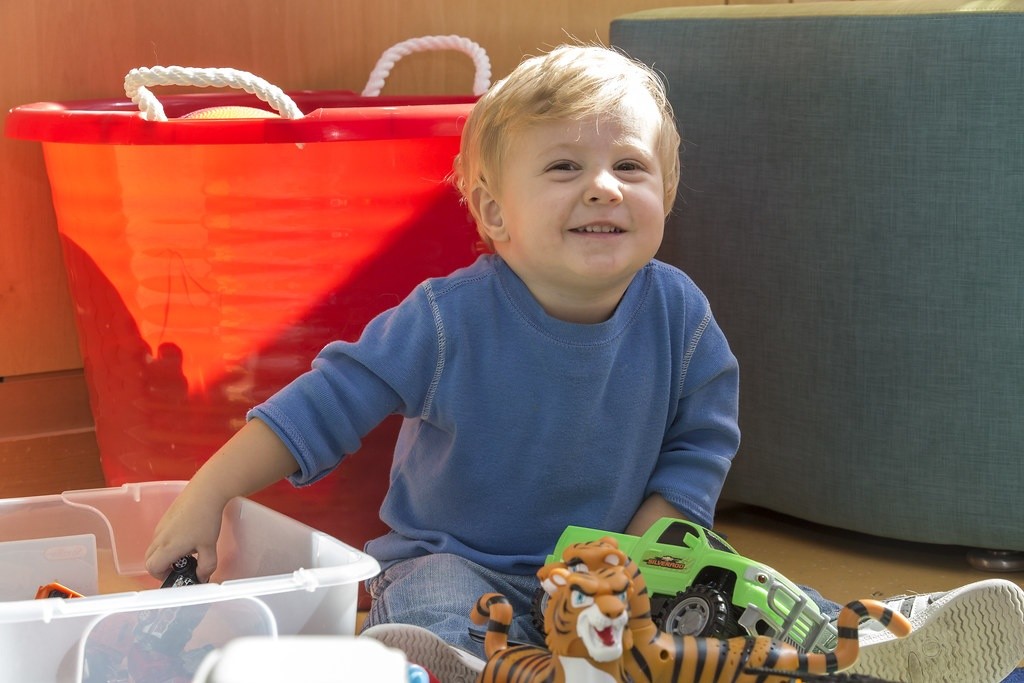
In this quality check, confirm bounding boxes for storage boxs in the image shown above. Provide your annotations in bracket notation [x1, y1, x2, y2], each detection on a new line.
[0, 482, 384, 683]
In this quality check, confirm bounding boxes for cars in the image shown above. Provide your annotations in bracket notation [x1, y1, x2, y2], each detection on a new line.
[132, 555, 214, 659]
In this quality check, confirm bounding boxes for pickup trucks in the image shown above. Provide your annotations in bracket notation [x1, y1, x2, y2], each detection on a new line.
[529, 516, 838, 654]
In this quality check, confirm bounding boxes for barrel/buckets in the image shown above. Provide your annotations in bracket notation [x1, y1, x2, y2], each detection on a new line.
[4, 33, 501, 614]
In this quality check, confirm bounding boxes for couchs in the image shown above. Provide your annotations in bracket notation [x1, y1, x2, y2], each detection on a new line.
[607, 0, 1024, 575]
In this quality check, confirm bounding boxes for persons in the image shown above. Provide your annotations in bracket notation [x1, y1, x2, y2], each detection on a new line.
[145, 44, 1024, 683]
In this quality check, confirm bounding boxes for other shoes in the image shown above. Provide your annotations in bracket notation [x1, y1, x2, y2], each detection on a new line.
[828, 579, 1022, 683]
[360, 624, 486, 683]
